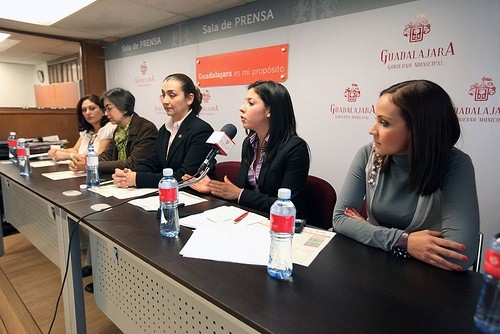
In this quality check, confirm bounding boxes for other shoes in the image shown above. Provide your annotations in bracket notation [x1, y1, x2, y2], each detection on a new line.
[85, 283, 94, 293]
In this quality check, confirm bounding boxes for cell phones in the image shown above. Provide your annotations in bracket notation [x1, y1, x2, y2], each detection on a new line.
[294, 219, 306, 233]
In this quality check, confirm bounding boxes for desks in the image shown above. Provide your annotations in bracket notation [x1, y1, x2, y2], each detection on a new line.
[0, 158, 113, 334]
[60, 186, 487, 334]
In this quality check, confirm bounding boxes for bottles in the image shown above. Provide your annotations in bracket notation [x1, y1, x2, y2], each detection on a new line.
[84, 145, 100, 189]
[473, 232, 500, 334]
[8, 132, 18, 165]
[158, 167, 181, 237]
[16, 138, 33, 176]
[267, 188, 296, 279]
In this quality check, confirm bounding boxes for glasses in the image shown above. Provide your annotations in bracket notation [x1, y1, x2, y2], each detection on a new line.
[103, 105, 116, 113]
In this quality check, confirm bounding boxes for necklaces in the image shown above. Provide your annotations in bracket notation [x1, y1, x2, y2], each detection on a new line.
[89, 133, 97, 145]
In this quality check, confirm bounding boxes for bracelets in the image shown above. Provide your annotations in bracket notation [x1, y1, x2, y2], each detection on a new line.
[71, 152, 73, 160]
[393, 231, 411, 261]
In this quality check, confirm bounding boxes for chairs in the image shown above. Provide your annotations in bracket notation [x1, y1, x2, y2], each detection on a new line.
[214, 161, 241, 183]
[305, 175, 336, 230]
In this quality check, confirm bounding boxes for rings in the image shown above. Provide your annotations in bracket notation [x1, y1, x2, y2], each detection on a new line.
[52, 157, 56, 160]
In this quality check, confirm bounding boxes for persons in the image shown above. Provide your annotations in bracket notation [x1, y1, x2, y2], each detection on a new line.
[47, 94, 117, 162]
[81, 73, 217, 293]
[68, 87, 158, 174]
[182, 79, 311, 212]
[333, 79, 479, 271]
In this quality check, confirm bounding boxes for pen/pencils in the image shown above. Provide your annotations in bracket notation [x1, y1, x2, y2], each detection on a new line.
[235, 210, 250, 222]
[100, 181, 114, 186]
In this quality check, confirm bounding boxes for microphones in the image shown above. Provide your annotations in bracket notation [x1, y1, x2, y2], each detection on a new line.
[197, 123, 237, 172]
